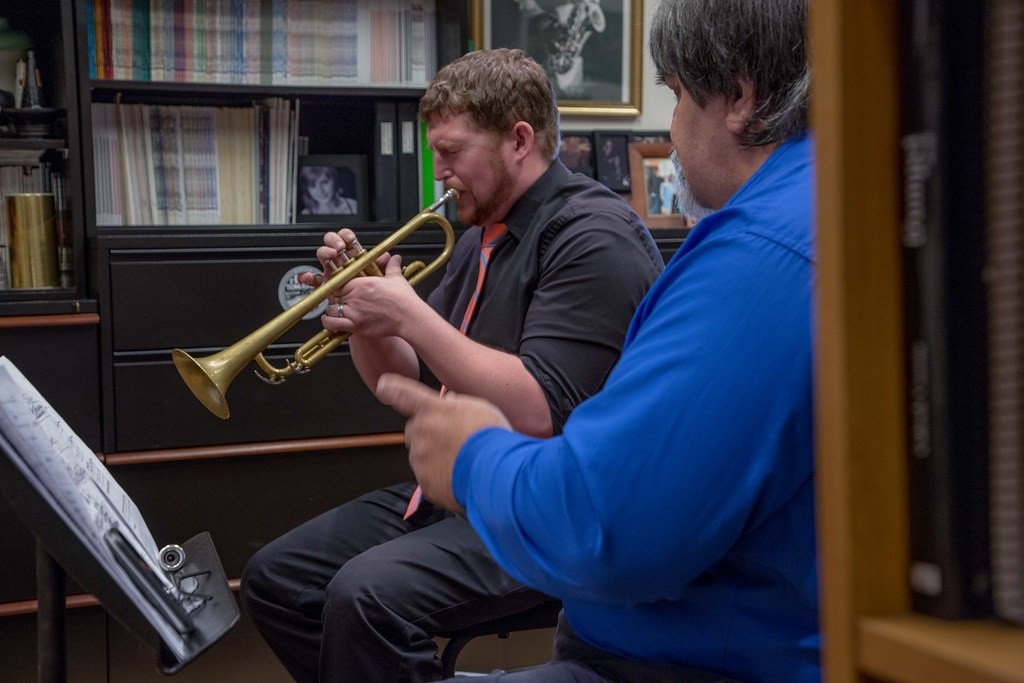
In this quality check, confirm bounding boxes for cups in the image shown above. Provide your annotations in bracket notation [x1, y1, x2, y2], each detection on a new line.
[7, 193, 58, 289]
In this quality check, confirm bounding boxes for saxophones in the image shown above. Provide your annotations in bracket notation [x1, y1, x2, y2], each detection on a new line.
[549, 0, 606, 75]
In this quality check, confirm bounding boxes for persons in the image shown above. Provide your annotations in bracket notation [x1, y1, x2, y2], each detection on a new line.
[302, 165, 358, 215]
[376, 0, 820, 683]
[647, 167, 677, 215]
[240, 47, 666, 683]
[0, 122, 72, 290]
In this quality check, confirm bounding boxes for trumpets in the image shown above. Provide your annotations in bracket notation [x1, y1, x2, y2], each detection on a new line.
[172, 187, 460, 420]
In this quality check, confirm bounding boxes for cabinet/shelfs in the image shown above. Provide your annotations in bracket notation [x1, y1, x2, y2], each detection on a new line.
[75, 2, 470, 256]
[1, 0, 97, 317]
[1, 314, 108, 683]
[805, 1, 1024, 683]
[90, 228, 691, 682]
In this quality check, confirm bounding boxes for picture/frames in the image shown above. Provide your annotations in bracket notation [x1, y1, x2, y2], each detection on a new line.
[296, 155, 365, 222]
[472, 0, 696, 229]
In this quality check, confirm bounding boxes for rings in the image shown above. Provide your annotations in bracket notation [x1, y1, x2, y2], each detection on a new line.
[338, 304, 344, 317]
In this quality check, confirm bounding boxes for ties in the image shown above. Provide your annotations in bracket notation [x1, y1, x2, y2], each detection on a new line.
[401, 222, 509, 522]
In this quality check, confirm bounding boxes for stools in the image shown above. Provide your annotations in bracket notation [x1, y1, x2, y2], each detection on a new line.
[434, 604, 561, 678]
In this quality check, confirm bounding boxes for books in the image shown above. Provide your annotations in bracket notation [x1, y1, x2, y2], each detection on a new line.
[85, 0, 475, 88]
[90, 96, 300, 228]
[373, 102, 459, 222]
